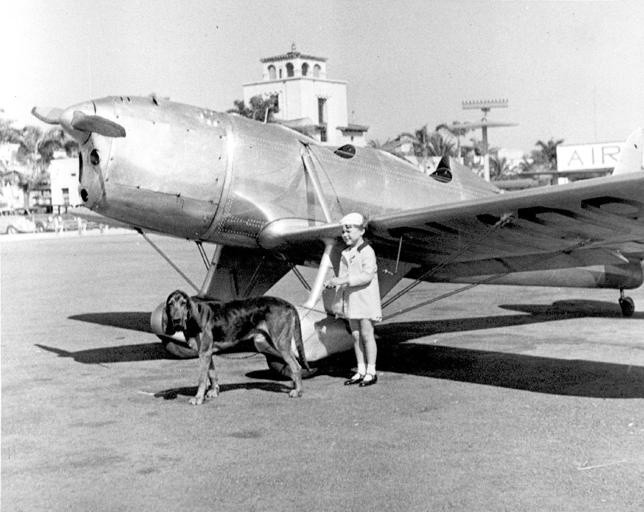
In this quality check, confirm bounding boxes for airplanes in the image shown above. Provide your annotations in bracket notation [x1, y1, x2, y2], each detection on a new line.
[32, 97, 643, 379]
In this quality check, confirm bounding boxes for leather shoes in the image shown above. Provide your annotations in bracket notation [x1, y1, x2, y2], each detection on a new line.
[360, 373, 377, 387]
[344, 374, 363, 385]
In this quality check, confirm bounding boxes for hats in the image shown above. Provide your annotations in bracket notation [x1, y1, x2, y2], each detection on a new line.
[341, 213, 367, 227]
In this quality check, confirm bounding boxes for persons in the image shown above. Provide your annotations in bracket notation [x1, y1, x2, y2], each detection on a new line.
[321, 210, 384, 387]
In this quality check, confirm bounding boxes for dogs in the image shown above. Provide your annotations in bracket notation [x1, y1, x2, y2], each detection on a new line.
[164, 289, 319, 408]
